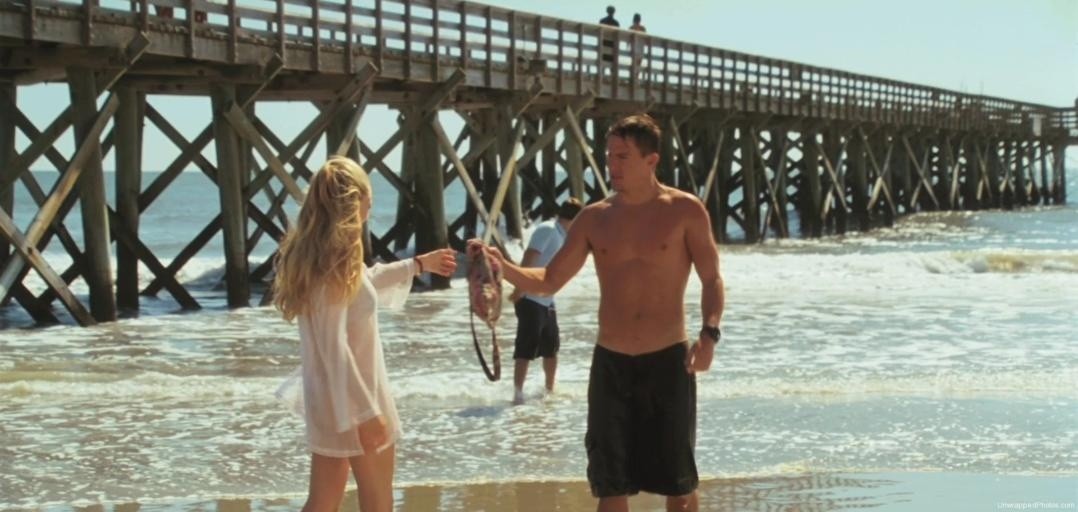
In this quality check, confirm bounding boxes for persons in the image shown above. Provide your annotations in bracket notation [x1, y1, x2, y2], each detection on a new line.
[599, 5, 620, 75]
[507, 198, 587, 390]
[272, 156, 458, 512]
[466, 115, 725, 512]
[626, 13, 646, 78]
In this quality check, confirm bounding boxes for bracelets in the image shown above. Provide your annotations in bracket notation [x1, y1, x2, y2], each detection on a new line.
[703, 324, 720, 341]
[414, 258, 423, 274]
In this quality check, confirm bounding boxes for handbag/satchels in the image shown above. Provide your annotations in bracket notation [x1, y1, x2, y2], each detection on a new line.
[466, 247, 502, 381]
